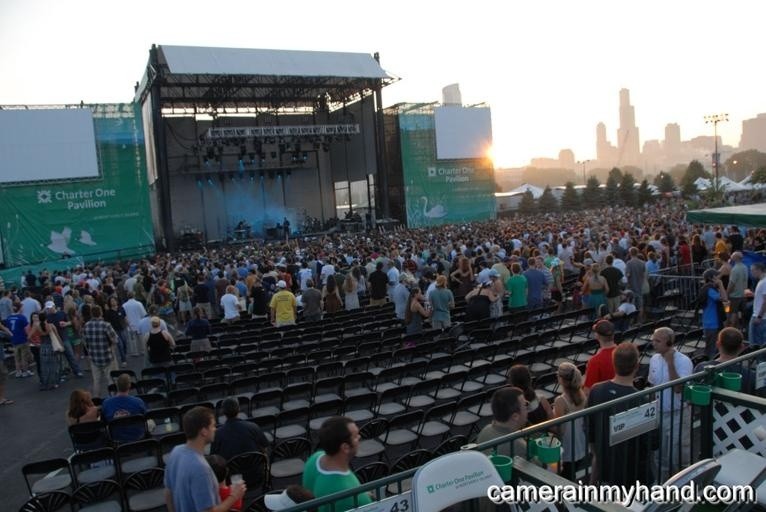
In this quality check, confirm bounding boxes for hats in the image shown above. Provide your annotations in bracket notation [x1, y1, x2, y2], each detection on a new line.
[264, 489, 307, 512]
[619, 289, 633, 298]
[704, 269, 720, 279]
[489, 269, 499, 277]
[592, 319, 615, 335]
[45, 301, 54, 308]
[276, 280, 286, 287]
[436, 275, 445, 286]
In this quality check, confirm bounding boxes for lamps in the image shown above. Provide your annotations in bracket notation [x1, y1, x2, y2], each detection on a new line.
[187, 131, 356, 187]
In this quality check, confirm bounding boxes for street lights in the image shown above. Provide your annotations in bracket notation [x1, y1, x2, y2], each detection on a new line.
[703, 113, 728, 198]
[577, 160, 590, 185]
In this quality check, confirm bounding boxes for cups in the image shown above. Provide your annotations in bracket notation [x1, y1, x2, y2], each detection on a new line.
[230, 474, 243, 485]
[723, 300, 732, 313]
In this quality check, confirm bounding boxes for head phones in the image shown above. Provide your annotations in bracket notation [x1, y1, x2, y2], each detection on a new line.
[666, 334, 673, 346]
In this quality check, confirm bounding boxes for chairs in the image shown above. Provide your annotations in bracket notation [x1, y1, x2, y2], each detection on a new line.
[13, 268, 758, 512]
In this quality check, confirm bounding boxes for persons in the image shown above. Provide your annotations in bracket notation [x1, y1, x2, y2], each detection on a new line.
[1, 191, 765, 509]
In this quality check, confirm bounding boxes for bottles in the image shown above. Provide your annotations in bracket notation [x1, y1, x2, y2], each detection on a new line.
[163, 417, 171, 433]
